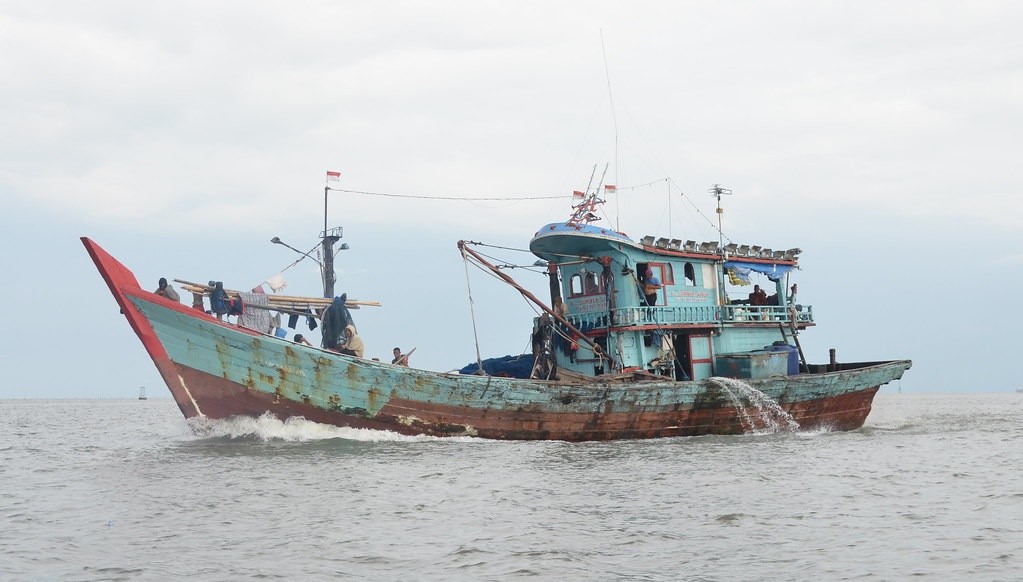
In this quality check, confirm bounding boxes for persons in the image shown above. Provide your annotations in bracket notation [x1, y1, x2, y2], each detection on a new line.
[749, 284, 769, 320]
[674, 334, 688, 380]
[294, 334, 313, 346]
[392, 347, 408, 366]
[153, 277, 180, 302]
[637, 268, 661, 306]
[553, 296, 569, 315]
[532, 357, 561, 381]
[338, 325, 364, 357]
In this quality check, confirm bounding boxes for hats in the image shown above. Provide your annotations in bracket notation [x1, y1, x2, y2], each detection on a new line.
[645, 269, 652, 275]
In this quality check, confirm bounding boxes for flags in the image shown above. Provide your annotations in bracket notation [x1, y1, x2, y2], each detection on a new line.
[327, 171, 341, 182]
[573, 190, 584, 199]
[605, 185, 615, 193]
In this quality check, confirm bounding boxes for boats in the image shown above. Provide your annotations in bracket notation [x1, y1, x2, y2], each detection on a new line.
[80, 159, 912, 443]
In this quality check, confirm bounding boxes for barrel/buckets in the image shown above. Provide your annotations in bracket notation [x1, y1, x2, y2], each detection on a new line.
[766, 341, 800, 375]
